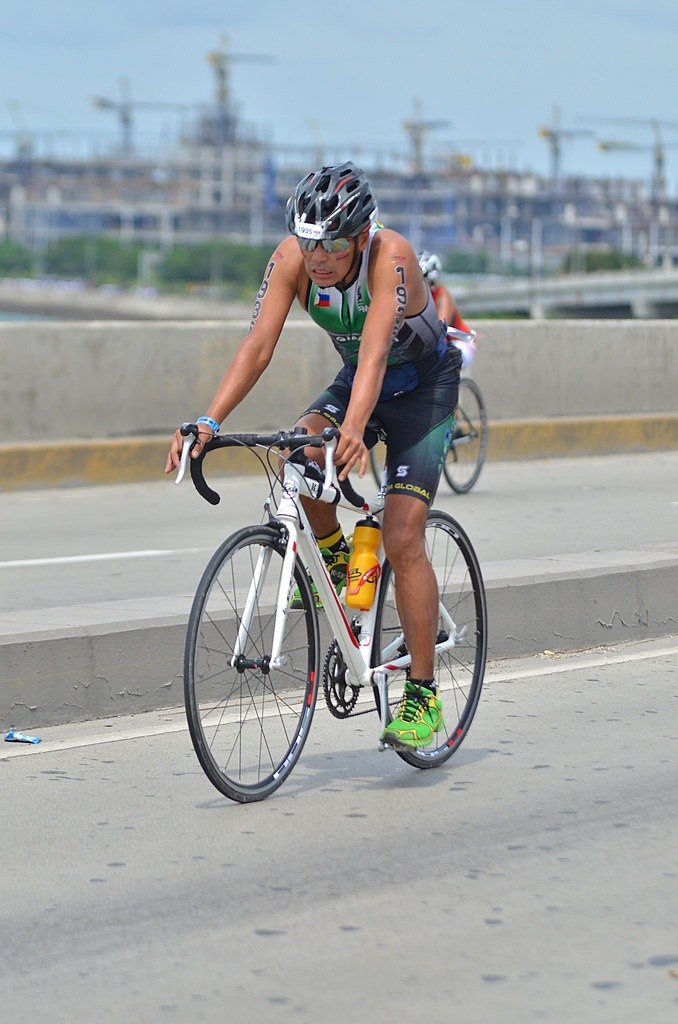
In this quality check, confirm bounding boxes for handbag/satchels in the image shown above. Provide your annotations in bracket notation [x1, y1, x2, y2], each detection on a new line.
[343, 318, 448, 402]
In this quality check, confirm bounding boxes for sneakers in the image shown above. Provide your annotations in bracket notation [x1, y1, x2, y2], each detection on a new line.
[289, 539, 353, 612]
[380, 681, 444, 747]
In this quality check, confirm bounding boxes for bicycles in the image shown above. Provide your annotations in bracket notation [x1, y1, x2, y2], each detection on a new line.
[170, 422, 489, 804]
[364, 376, 488, 494]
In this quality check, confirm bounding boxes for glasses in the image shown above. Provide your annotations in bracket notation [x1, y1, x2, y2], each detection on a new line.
[296, 237, 355, 254]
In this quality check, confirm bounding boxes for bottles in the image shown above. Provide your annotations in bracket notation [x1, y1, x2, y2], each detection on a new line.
[346, 515, 383, 610]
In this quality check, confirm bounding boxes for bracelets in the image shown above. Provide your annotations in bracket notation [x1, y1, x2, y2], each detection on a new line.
[196, 417, 220, 435]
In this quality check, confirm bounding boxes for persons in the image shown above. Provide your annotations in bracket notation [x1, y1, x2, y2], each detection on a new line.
[415, 250, 477, 444]
[166, 161, 464, 747]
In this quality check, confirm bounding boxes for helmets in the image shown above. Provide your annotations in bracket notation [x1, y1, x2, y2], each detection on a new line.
[285, 161, 378, 241]
[418, 250, 442, 282]
